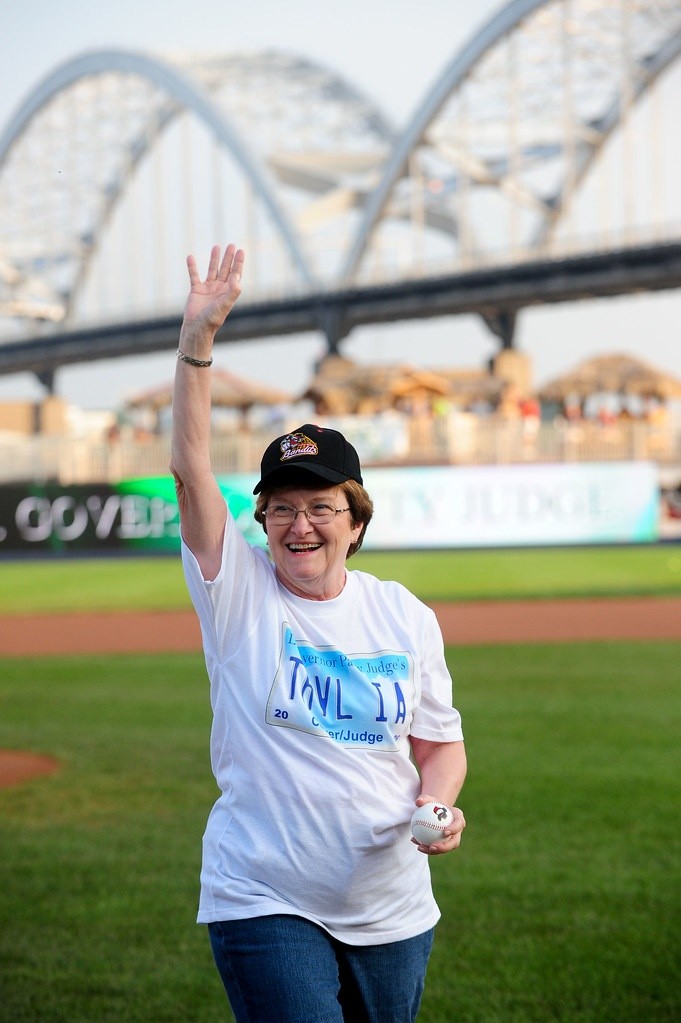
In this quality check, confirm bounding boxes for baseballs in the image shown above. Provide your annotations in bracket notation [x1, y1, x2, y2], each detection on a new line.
[410, 801, 455, 847]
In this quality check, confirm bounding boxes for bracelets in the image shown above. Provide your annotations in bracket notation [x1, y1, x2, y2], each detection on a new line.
[175, 349, 215, 367]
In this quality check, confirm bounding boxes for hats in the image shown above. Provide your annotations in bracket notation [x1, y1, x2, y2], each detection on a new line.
[253, 424, 363, 485]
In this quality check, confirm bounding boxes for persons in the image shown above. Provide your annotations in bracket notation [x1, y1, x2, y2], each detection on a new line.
[167, 246, 469, 1023]
[519, 394, 538, 424]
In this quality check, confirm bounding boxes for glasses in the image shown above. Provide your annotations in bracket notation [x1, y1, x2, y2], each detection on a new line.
[262, 502, 351, 525]
